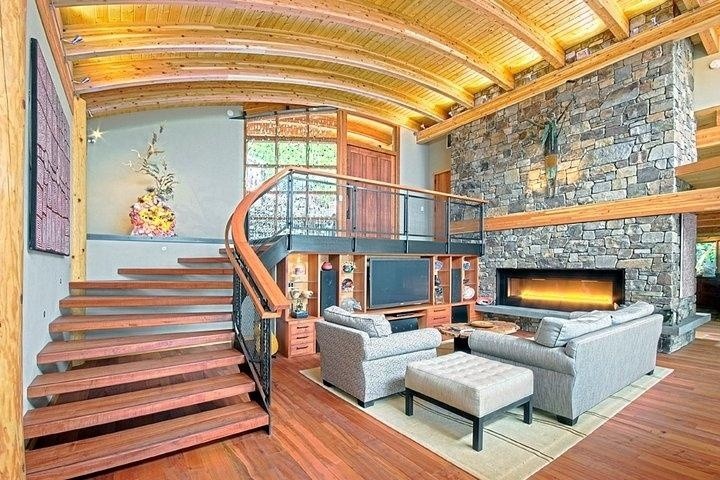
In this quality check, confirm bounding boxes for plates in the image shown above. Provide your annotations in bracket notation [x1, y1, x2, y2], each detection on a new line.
[470, 320, 496, 328]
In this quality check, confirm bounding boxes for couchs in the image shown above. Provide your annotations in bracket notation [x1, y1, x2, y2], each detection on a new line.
[467, 300, 665, 426]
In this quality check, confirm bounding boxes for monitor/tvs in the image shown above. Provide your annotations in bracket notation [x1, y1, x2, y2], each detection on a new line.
[369, 258, 430, 309]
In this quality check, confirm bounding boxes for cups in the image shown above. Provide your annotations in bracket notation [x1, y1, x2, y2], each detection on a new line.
[290, 287, 313, 298]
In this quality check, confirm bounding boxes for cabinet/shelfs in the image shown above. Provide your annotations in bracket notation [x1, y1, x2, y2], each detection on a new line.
[329, 254, 366, 314]
[416, 253, 482, 342]
[276, 251, 321, 359]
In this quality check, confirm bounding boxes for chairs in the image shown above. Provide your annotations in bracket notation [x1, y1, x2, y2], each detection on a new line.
[314, 304, 443, 408]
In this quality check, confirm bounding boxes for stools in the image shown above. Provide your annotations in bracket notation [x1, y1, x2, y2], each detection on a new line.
[402, 351, 535, 452]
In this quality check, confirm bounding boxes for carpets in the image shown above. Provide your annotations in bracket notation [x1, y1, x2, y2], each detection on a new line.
[298, 336, 674, 480]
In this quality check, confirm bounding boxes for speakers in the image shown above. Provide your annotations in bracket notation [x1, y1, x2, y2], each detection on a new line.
[451, 268, 461, 302]
[321, 271, 336, 316]
[452, 306, 468, 323]
